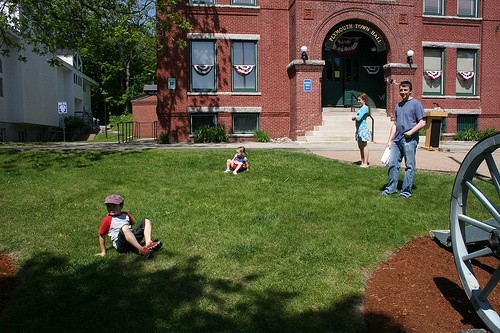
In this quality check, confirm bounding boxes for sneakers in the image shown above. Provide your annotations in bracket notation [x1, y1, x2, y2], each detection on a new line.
[147, 241, 163, 252]
[140, 247, 153, 257]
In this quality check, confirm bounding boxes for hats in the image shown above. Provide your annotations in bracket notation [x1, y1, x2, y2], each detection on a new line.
[104, 194, 124, 205]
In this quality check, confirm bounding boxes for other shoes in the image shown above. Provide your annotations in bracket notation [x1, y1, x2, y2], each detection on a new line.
[224, 170, 230, 174]
[232, 171, 237, 175]
[360, 165, 369, 168]
[398, 192, 412, 199]
[378, 191, 388, 196]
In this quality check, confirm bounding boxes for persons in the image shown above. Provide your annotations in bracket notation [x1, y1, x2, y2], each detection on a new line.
[351, 93, 372, 167]
[382, 80, 427, 196]
[95, 193, 162, 257]
[225, 146, 250, 175]
